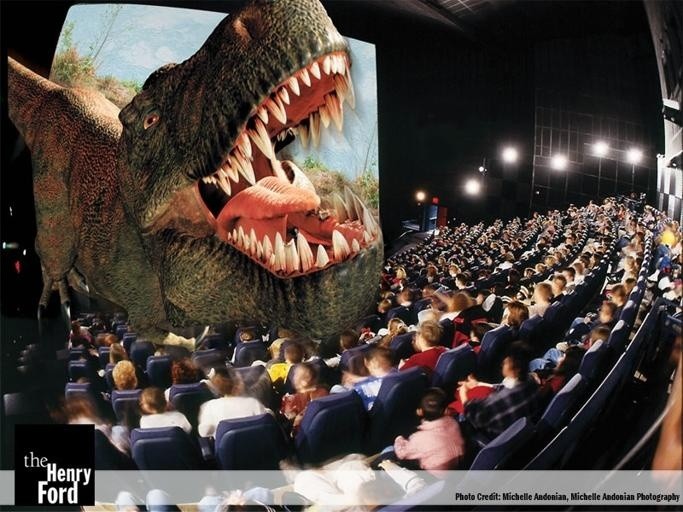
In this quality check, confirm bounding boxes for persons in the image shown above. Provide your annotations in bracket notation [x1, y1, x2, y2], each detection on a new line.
[68, 192, 683, 510]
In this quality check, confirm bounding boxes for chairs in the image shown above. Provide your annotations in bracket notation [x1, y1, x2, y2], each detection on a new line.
[3, 202, 682, 511]
